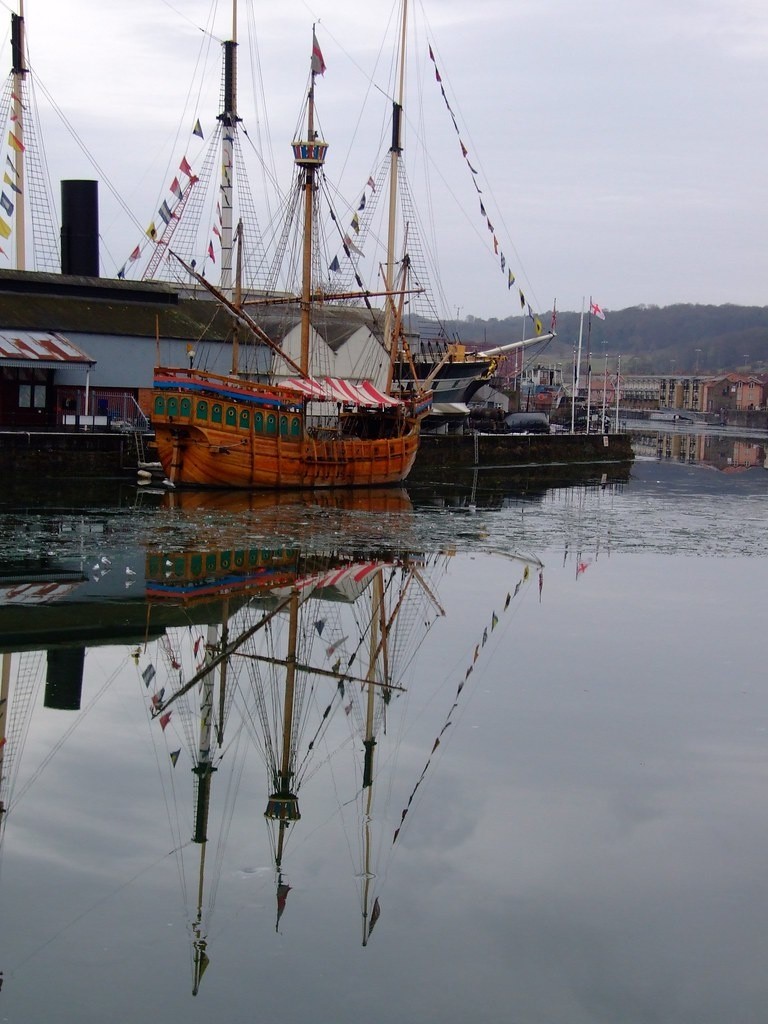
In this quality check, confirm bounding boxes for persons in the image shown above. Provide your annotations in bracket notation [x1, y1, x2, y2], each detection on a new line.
[605, 415, 610, 433]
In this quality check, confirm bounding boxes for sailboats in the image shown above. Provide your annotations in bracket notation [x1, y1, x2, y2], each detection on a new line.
[135, 487, 547, 998]
[137, 0, 442, 497]
[230, 0, 565, 429]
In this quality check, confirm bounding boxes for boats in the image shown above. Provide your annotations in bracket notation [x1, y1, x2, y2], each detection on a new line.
[0, 330, 138, 489]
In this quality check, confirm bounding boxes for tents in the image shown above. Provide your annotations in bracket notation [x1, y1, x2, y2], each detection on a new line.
[289, 561, 396, 592]
[278, 377, 399, 409]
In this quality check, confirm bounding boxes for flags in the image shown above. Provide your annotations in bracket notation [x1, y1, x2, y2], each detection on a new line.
[312, 34, 326, 74]
[314, 616, 353, 716]
[0, 93, 24, 238]
[392, 566, 533, 847]
[539, 574, 542, 599]
[578, 560, 592, 573]
[575, 361, 620, 386]
[551, 309, 556, 332]
[118, 120, 233, 280]
[590, 304, 605, 320]
[328, 177, 375, 274]
[428, 46, 542, 336]
[133, 638, 217, 768]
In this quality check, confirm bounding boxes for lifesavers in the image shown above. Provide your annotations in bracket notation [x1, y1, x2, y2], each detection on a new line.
[538, 394, 544, 400]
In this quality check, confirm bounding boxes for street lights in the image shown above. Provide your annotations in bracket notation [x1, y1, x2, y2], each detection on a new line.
[694, 348, 701, 371]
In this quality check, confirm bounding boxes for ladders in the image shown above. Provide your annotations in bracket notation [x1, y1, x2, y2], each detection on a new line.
[134, 430, 146, 466]
